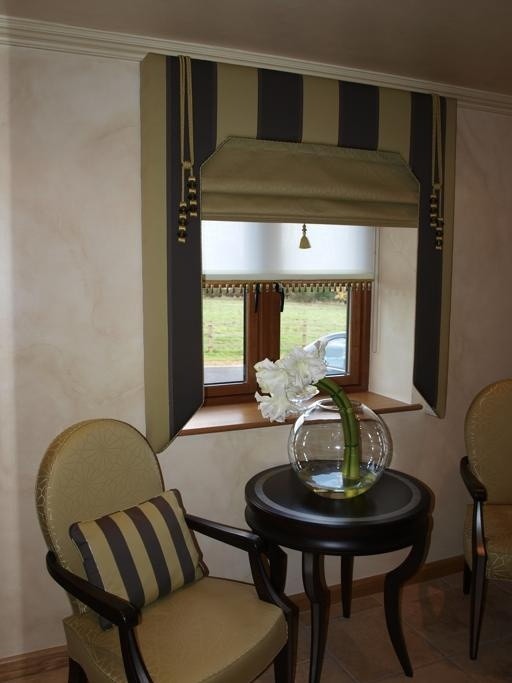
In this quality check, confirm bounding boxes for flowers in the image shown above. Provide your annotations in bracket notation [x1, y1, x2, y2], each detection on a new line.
[251, 345, 364, 487]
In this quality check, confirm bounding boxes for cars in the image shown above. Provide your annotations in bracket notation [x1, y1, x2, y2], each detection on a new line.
[303, 333, 346, 376]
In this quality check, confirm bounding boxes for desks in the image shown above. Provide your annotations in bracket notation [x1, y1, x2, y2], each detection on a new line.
[246, 463, 427, 674]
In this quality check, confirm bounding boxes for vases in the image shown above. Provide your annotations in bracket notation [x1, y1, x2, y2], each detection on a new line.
[285, 393, 394, 500]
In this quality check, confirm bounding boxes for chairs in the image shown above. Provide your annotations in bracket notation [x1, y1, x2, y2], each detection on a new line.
[35, 421, 304, 675]
[458, 375, 511, 645]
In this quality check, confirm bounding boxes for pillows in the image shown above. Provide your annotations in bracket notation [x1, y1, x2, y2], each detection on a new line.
[68, 487, 212, 633]
